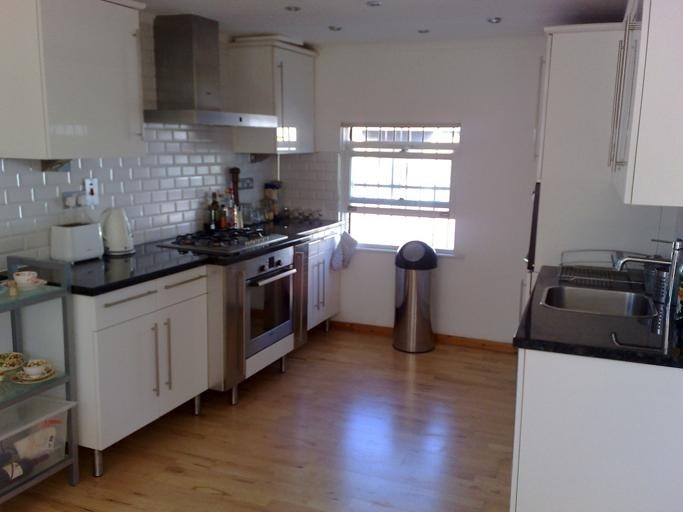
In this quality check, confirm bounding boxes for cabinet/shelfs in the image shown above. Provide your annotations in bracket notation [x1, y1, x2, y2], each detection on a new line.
[1, 267, 210, 477]
[605, 1, 683, 208]
[510, 348, 683, 512]
[1, 0, 147, 162]
[232, 39, 318, 155]
[529, 23, 663, 272]
[0, 254, 80, 505]
[518, 271, 539, 316]
[299, 220, 344, 333]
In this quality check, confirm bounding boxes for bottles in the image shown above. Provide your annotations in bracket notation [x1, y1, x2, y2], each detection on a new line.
[210, 192, 219, 230]
[284, 208, 288, 229]
[229, 186, 234, 199]
[220, 211, 226, 227]
[225, 188, 230, 204]
[229, 200, 235, 230]
[221, 204, 227, 230]
[0, 453, 49, 487]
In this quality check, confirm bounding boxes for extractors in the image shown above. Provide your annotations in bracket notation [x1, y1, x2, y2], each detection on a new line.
[143, 13, 277, 129]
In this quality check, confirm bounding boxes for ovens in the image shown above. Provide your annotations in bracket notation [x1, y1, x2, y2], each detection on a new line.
[244, 247, 297, 359]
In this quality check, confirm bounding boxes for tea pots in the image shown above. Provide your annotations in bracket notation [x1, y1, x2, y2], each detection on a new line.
[98, 205, 137, 256]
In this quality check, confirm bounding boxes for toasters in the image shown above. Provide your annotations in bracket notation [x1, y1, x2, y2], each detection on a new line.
[49, 222, 104, 265]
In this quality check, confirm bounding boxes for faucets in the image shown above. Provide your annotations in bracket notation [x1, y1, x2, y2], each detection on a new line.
[610, 308, 675, 357]
[613, 237, 683, 307]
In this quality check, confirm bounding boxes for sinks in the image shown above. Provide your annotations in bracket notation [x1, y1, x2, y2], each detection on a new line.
[539, 284, 658, 320]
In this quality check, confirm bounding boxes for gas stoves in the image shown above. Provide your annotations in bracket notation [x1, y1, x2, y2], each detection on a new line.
[163, 227, 288, 254]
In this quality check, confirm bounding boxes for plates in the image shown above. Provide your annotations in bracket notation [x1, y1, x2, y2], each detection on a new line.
[9, 371, 56, 383]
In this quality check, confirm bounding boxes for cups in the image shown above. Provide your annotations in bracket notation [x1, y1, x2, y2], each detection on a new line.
[13, 271, 36, 286]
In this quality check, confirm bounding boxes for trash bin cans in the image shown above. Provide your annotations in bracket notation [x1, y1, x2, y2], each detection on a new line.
[392, 239, 438, 354]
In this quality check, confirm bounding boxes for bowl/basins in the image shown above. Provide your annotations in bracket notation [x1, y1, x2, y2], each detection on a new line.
[22, 359, 45, 376]
